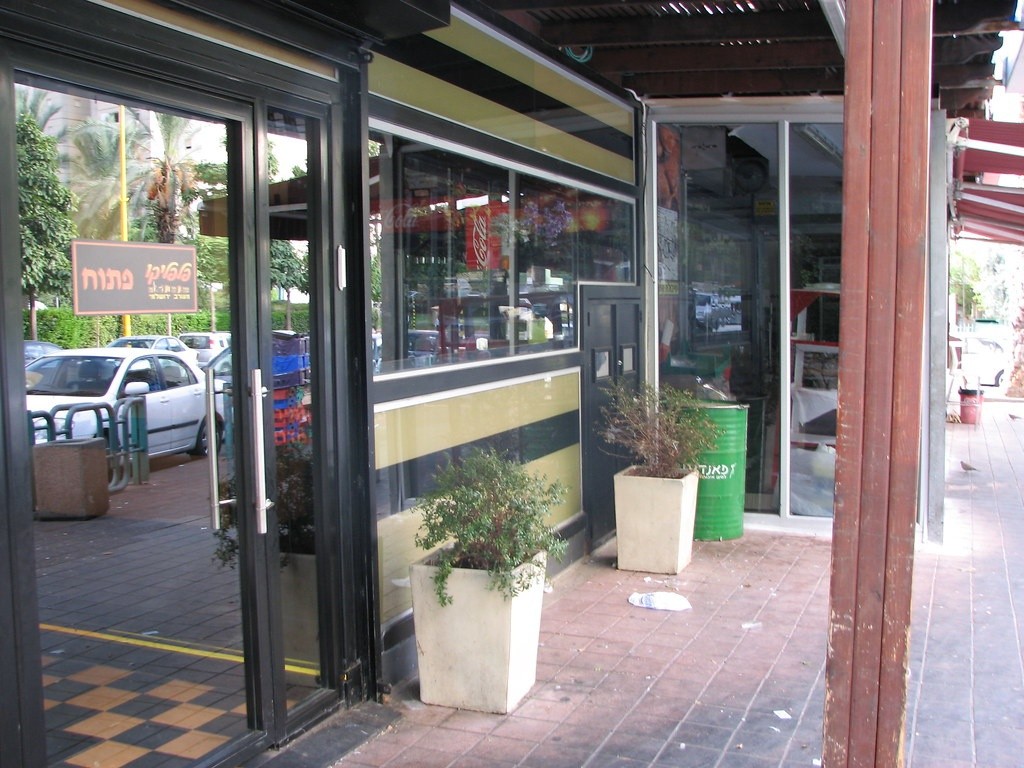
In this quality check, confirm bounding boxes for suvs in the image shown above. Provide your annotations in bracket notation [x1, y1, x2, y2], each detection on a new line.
[178, 331, 232, 375]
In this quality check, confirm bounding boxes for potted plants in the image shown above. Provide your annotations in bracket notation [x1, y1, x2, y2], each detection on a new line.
[599, 376, 726, 574]
[408, 447, 566, 712]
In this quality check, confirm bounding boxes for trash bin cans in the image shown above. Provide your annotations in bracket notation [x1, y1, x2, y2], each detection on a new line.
[959, 386, 985, 424]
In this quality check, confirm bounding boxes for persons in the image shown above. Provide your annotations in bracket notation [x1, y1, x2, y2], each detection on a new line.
[122, 341, 133, 347]
[101, 366, 127, 400]
[458, 325, 464, 339]
[434, 316, 440, 332]
[371, 326, 383, 373]
[137, 341, 147, 348]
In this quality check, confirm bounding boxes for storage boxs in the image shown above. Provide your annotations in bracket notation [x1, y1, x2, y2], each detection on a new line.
[271, 337, 313, 448]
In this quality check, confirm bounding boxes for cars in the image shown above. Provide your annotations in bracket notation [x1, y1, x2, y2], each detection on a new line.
[407, 330, 440, 368]
[105, 335, 200, 378]
[695, 292, 718, 323]
[24, 347, 233, 483]
[24, 340, 64, 368]
[199, 346, 233, 383]
[962, 336, 1006, 387]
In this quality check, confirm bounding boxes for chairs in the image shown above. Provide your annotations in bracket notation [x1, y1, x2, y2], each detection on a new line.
[69, 363, 101, 389]
[164, 365, 182, 389]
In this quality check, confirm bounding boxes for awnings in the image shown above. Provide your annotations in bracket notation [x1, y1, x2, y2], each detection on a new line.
[946, 114, 1024, 248]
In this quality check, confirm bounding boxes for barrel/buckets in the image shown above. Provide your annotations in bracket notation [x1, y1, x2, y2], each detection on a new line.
[666, 399, 749, 541]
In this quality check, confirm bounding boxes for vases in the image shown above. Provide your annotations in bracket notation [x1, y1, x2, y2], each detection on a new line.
[33, 438, 109, 521]
